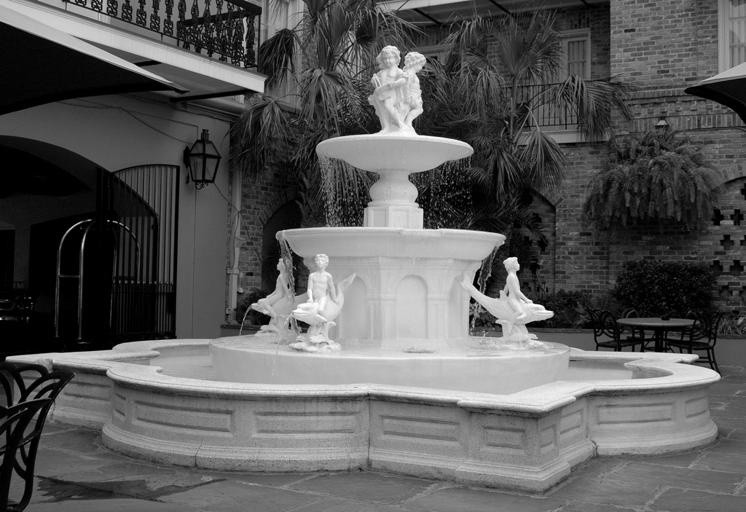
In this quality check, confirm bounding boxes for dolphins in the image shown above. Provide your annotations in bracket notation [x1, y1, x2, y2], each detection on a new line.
[292, 273, 357, 327]
[251, 292, 309, 317]
[455, 269, 554, 325]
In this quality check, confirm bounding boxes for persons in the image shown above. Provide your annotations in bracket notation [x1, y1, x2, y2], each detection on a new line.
[306, 253, 339, 313]
[257, 257, 293, 319]
[370, 45, 426, 133]
[503, 257, 533, 321]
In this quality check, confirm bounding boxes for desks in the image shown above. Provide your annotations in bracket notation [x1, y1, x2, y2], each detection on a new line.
[617, 317, 699, 352]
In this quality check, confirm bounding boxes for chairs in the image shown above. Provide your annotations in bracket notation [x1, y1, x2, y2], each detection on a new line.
[0, 358, 75, 512]
[619, 307, 646, 352]
[667, 311, 722, 376]
[586, 306, 636, 351]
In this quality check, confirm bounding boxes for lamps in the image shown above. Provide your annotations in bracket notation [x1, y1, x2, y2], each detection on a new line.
[184, 129, 220, 190]
